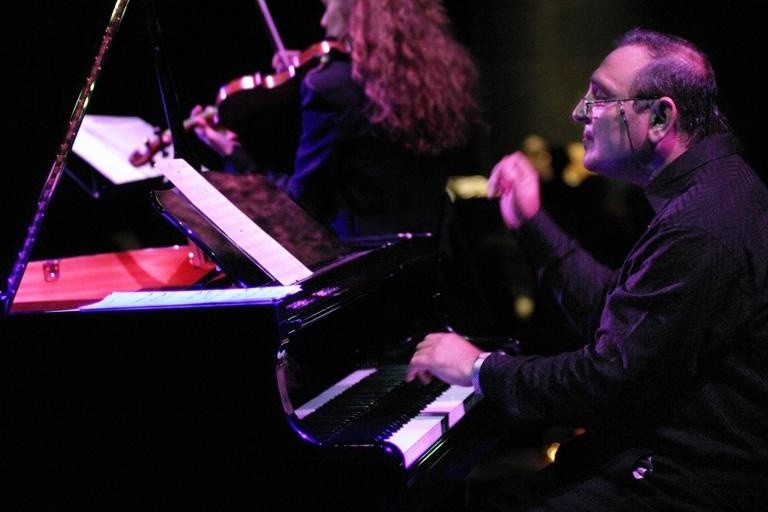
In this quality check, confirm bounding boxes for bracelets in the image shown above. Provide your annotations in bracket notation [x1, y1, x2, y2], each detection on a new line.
[472, 351, 491, 396]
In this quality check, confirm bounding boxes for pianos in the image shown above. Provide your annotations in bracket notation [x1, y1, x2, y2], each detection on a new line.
[0, 238, 527, 508]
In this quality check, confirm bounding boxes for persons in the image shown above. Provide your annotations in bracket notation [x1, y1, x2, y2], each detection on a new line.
[181, 0, 480, 241]
[402, 28, 767, 510]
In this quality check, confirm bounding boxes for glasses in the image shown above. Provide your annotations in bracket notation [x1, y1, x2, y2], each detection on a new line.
[577, 93, 660, 115]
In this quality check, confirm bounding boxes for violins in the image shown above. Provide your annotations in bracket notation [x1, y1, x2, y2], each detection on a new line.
[129, 42, 350, 167]
